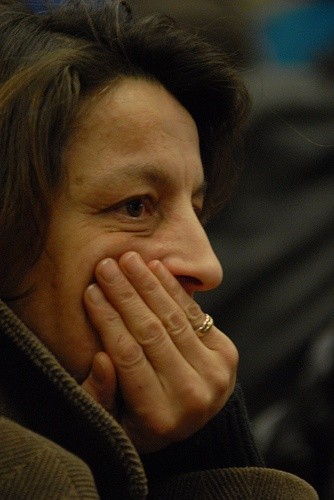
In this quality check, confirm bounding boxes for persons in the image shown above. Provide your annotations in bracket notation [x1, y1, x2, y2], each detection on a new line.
[0, 0, 326, 500]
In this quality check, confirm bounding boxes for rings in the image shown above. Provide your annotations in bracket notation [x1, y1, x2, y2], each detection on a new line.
[194, 314, 215, 337]
